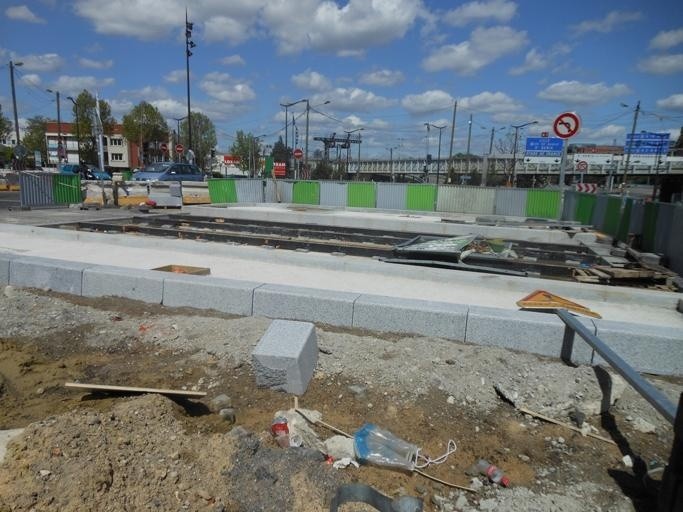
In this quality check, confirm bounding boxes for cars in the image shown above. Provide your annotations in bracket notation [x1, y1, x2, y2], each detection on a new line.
[61, 162, 110, 180]
[130, 161, 205, 182]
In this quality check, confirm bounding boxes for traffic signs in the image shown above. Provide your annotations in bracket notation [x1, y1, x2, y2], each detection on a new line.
[625, 140, 670, 147]
[525, 137, 563, 155]
[624, 147, 669, 154]
[625, 133, 670, 140]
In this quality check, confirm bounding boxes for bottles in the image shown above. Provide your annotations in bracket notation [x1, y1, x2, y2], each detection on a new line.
[271, 415, 291, 450]
[476, 459, 509, 486]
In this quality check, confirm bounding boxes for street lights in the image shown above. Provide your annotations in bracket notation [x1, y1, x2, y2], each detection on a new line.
[420, 121, 447, 184]
[173, 114, 188, 146]
[280, 99, 363, 170]
[509, 120, 540, 187]
[487, 125, 507, 153]
[183, 7, 196, 150]
[65, 95, 81, 165]
[45, 84, 60, 166]
[618, 100, 642, 188]
[8, 60, 23, 147]
[384, 144, 397, 181]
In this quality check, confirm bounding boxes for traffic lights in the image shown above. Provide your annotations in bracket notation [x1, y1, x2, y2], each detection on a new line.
[210, 145, 215, 159]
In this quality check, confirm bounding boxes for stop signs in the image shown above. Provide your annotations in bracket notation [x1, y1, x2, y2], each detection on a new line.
[175, 144, 184, 154]
[159, 142, 168, 152]
